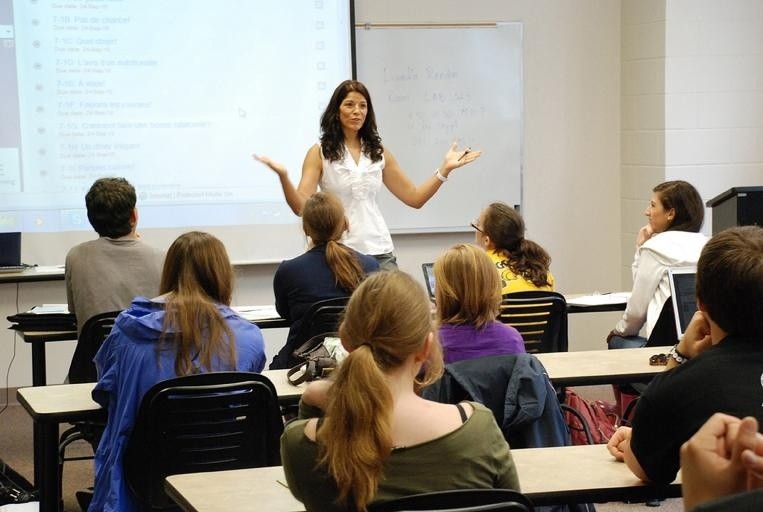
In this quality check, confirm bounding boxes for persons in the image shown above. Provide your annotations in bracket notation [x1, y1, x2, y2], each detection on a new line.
[75, 230, 266, 511]
[64, 176, 169, 383]
[426, 243, 525, 376]
[268, 191, 381, 371]
[607, 180, 711, 423]
[679, 411, 763, 512]
[607, 225, 763, 485]
[280, 271, 522, 512]
[471, 201, 554, 356]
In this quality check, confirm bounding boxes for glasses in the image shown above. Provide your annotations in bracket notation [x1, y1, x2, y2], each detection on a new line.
[470, 216, 489, 238]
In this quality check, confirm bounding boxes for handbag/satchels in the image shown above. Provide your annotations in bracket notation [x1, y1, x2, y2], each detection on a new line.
[562, 388, 620, 444]
[285, 331, 350, 385]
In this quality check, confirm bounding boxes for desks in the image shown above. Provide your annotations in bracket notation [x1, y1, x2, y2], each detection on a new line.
[163, 436, 682, 512]
[17, 342, 683, 512]
[16, 290, 635, 384]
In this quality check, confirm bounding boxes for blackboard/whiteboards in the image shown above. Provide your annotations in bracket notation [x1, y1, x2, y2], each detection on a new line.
[355, 21, 524, 234]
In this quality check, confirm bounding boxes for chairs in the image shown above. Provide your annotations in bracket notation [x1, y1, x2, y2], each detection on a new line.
[646, 291, 700, 348]
[362, 489, 534, 511]
[59, 309, 125, 478]
[76, 374, 285, 512]
[492, 291, 569, 355]
[286, 298, 350, 367]
[559, 397, 641, 445]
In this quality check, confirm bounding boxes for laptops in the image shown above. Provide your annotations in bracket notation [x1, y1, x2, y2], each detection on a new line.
[0, 231, 22, 266]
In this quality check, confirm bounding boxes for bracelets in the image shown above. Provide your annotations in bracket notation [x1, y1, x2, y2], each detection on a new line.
[252, 80, 481, 272]
[669, 344, 688, 365]
[435, 168, 447, 182]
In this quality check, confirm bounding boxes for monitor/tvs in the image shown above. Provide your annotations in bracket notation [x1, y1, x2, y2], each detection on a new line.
[422, 262, 443, 306]
[668, 266, 706, 344]
